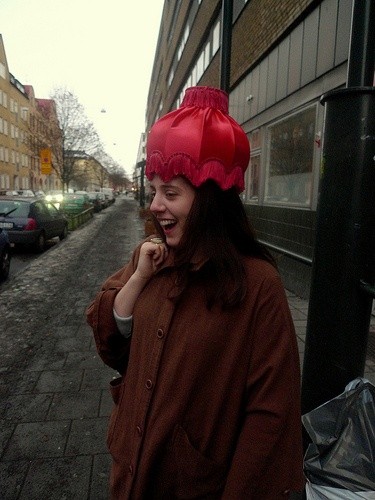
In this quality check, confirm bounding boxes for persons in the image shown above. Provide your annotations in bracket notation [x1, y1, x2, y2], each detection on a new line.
[84, 86, 307, 500]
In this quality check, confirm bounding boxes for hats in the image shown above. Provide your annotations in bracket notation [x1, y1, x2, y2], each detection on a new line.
[146, 86, 250, 190]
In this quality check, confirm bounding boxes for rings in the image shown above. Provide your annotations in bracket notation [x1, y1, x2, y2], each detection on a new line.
[150, 237, 165, 244]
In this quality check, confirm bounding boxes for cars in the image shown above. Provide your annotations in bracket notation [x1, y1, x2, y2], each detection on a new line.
[59, 193, 88, 215]
[45, 190, 62, 203]
[33, 189, 48, 199]
[88, 192, 102, 211]
[0, 196, 70, 249]
[0, 228, 12, 280]
[0, 189, 35, 198]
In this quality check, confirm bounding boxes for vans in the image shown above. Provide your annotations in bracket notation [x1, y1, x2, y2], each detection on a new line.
[100, 187, 114, 203]
